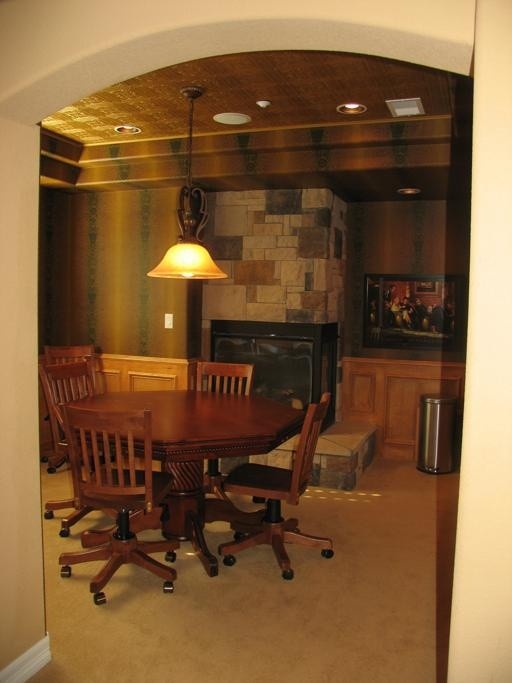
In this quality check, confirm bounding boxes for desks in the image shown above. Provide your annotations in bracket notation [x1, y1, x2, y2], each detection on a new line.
[68, 388, 307, 575]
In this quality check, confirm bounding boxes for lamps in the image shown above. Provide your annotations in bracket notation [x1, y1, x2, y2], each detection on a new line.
[145, 85, 234, 283]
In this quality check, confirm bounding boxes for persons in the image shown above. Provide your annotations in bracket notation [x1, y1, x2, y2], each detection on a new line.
[370, 284, 455, 334]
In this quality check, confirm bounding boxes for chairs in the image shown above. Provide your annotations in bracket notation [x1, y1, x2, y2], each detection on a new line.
[38, 355, 134, 539]
[57, 405, 180, 603]
[47, 343, 113, 476]
[184, 361, 265, 533]
[223, 390, 335, 581]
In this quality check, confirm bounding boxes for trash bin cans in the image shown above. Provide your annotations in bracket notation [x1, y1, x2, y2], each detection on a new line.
[416, 394, 458, 474]
